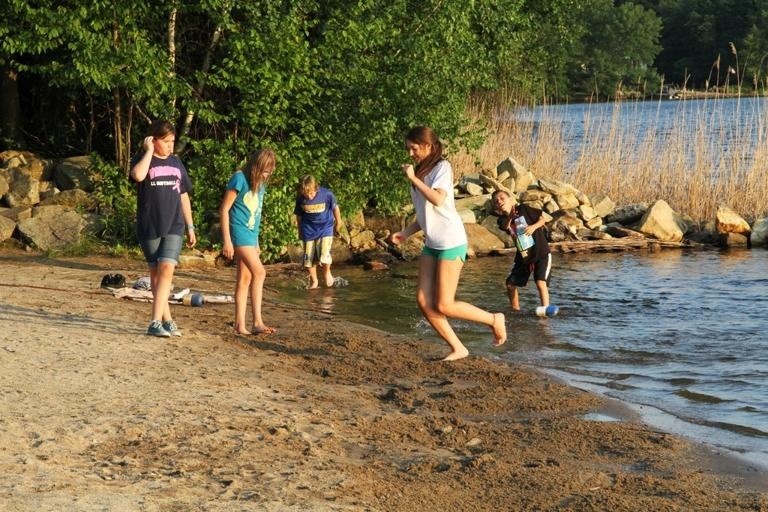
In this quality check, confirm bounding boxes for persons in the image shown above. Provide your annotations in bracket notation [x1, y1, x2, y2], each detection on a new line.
[293, 173, 342, 291]
[129, 120, 197, 338]
[217, 147, 276, 335]
[389, 125, 507, 361]
[491, 188, 552, 312]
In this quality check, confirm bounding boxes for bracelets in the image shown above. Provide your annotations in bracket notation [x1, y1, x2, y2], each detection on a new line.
[185, 223, 195, 230]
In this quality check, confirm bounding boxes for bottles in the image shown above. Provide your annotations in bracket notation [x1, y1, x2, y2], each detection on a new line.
[183, 291, 205, 308]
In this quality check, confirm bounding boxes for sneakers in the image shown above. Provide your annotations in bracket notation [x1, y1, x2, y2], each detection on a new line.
[147, 319, 170, 338]
[161, 319, 182, 337]
